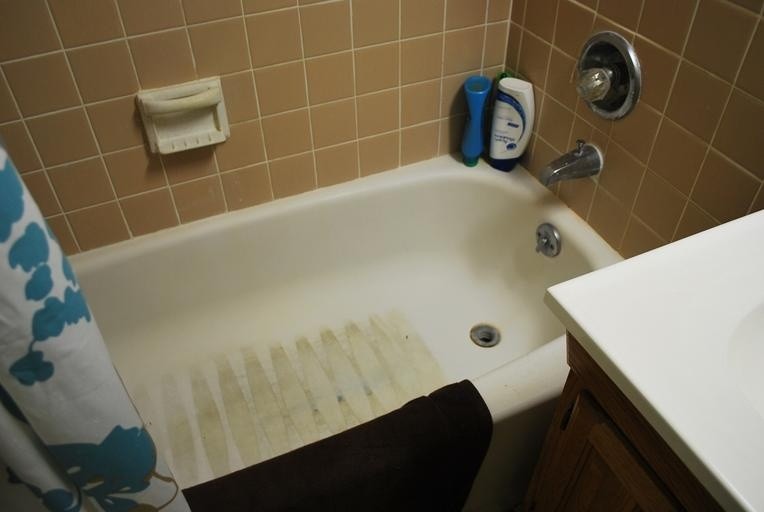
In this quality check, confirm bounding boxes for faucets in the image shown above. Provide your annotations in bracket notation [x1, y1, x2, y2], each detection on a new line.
[540, 141, 602, 187]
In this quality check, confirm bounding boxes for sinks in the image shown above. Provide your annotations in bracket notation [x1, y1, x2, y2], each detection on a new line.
[542, 211, 763, 512]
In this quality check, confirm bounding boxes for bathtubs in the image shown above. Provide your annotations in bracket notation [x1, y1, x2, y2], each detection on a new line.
[65, 150, 627, 511]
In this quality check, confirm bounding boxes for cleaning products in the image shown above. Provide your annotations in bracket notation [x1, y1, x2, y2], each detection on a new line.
[462, 74, 493, 167]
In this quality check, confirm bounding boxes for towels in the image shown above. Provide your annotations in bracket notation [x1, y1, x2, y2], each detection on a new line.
[179, 378, 495, 512]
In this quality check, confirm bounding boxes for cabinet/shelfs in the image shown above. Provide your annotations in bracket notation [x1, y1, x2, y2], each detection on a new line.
[518, 331, 724, 511]
